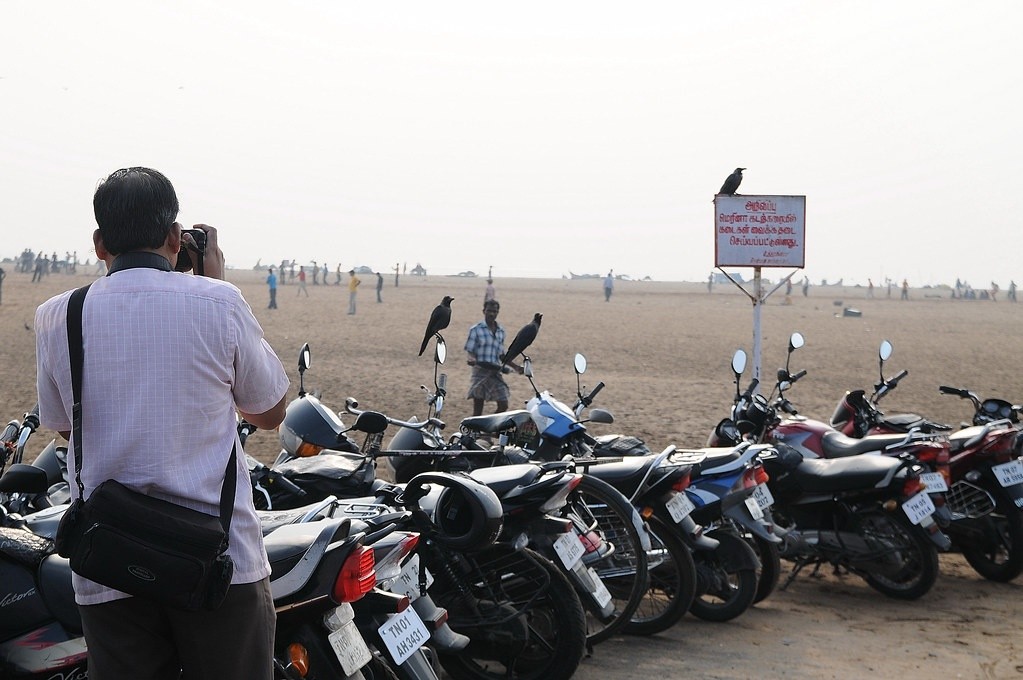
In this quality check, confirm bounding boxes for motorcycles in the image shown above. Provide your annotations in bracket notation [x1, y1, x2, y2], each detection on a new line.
[0, 329, 1023, 680]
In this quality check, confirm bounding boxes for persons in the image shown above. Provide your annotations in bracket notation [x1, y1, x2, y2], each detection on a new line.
[786, 280, 791, 294]
[313, 262, 342, 285]
[952, 278, 1017, 301]
[267, 269, 277, 309]
[392, 263, 399, 287]
[485, 279, 494, 301]
[18, 248, 77, 282]
[868, 278, 873, 287]
[802, 276, 809, 296]
[901, 279, 908, 300]
[603, 269, 614, 301]
[376, 272, 383, 303]
[96, 259, 107, 276]
[465, 299, 524, 416]
[347, 270, 361, 314]
[0, 268, 6, 305]
[34, 166, 291, 680]
[279, 260, 308, 297]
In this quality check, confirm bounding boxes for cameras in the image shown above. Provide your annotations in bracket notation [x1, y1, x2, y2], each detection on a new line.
[173, 228, 207, 272]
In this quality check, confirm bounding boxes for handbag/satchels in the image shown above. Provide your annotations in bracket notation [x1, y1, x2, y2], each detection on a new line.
[54, 479, 230, 610]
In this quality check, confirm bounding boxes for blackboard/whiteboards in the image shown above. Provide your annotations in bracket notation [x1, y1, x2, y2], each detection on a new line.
[715, 194, 806, 269]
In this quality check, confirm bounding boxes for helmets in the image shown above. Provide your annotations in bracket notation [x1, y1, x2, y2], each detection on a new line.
[403, 472, 504, 553]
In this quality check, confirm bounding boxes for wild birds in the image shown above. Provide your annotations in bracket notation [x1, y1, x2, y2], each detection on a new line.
[712, 167, 747, 204]
[418, 295, 455, 357]
[500, 312, 544, 371]
